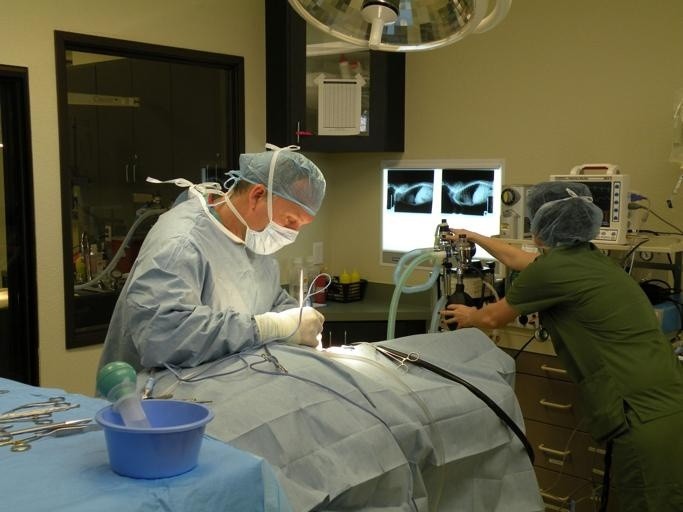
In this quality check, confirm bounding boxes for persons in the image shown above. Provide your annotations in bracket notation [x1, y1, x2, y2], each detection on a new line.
[93, 141, 326, 402]
[436, 179, 683, 512]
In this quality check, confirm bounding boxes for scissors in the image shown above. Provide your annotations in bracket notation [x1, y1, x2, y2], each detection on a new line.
[370, 344, 419, 374]
[0, 396, 91, 452]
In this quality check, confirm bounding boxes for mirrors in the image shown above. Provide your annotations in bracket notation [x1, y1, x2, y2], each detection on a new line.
[53, 28, 244, 353]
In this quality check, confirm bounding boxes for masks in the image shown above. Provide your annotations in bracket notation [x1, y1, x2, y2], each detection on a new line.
[240, 219, 300, 260]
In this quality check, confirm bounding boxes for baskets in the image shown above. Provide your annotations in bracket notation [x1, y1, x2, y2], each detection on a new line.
[323, 276, 368, 303]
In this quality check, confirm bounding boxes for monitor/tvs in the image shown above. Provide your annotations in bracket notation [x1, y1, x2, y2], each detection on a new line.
[377, 157, 506, 278]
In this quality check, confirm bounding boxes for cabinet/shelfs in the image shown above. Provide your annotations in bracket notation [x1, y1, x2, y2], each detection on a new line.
[491, 346, 612, 512]
[265, 0, 406, 153]
[321, 320, 436, 346]
[65, 58, 228, 221]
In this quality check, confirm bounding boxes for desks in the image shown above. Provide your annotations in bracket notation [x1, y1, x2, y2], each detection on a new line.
[0, 377, 292, 512]
